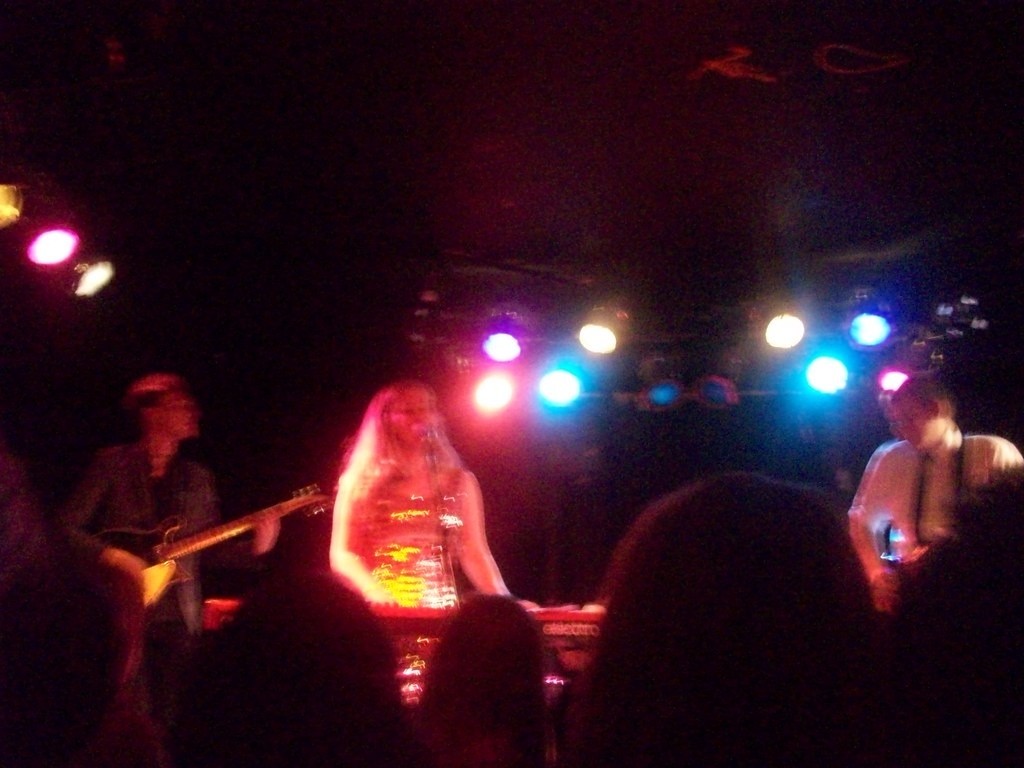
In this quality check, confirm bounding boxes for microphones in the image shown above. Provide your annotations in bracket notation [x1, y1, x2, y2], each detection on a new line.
[413, 423, 438, 441]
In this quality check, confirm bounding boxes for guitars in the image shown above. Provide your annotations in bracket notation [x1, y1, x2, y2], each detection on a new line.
[90, 482, 335, 615]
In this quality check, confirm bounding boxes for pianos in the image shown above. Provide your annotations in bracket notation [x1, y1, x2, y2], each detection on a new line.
[337, 601, 614, 651]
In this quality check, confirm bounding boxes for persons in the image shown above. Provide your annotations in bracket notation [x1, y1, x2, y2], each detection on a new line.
[848, 363, 1023, 608]
[0, 471, 1023, 768]
[327, 381, 538, 701]
[67, 373, 279, 641]
